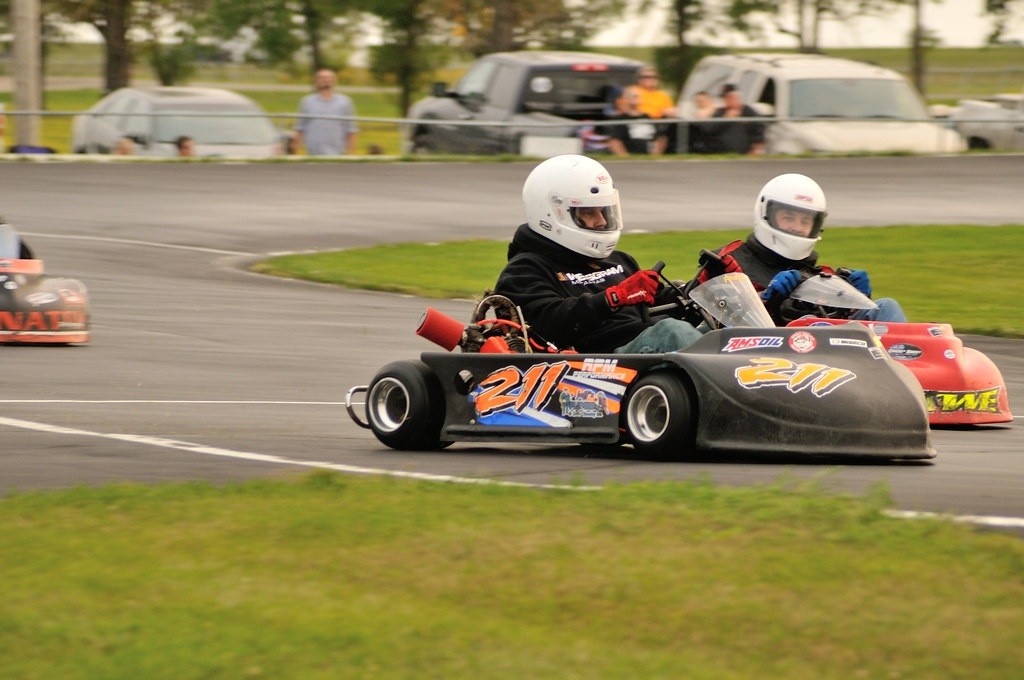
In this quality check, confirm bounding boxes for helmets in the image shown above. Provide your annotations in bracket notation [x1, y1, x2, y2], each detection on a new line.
[523, 154, 623, 259]
[753, 173, 826, 260]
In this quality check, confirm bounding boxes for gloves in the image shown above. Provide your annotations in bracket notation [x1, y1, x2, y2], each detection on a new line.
[761, 269, 802, 301]
[605, 270, 659, 307]
[846, 268, 871, 298]
[699, 254, 742, 284]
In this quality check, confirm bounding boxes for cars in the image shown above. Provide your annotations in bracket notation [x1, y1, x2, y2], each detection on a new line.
[72, 87, 283, 158]
[929, 93, 1024, 151]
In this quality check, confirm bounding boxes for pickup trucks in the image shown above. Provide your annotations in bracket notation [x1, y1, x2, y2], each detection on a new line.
[406, 50, 652, 157]
[677, 52, 969, 156]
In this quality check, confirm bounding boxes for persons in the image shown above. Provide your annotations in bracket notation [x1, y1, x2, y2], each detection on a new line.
[292, 70, 359, 155]
[624, 67, 673, 119]
[177, 136, 196, 156]
[688, 84, 765, 154]
[595, 85, 642, 155]
[703, 172, 906, 322]
[492, 154, 743, 354]
